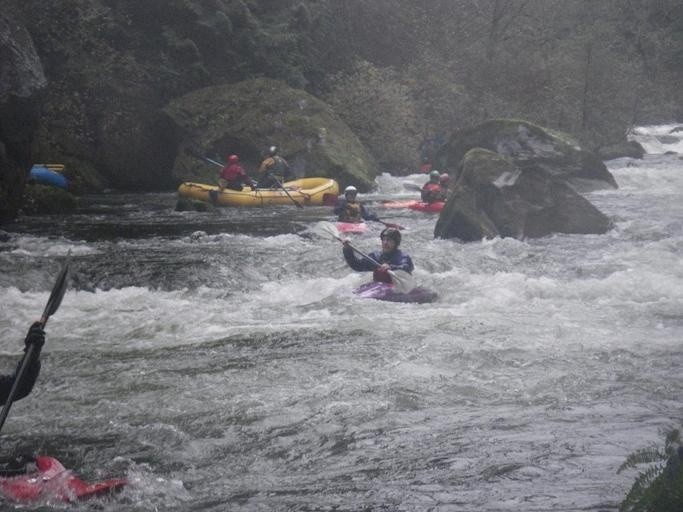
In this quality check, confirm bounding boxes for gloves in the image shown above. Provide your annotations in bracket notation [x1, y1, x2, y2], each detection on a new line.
[24, 320, 47, 347]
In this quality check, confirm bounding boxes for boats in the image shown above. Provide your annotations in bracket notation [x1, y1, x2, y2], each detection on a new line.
[25, 162, 78, 194]
[0, 453, 130, 504]
[348, 282, 437, 309]
[176, 175, 340, 212]
[378, 196, 448, 212]
[330, 219, 366, 238]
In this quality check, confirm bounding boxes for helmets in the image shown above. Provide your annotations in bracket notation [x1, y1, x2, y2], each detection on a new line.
[269, 146, 278, 155]
[229, 154, 238, 163]
[381, 227, 401, 246]
[430, 170, 448, 184]
[343, 186, 359, 195]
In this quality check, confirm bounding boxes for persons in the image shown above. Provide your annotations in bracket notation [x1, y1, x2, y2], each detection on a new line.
[219, 154, 254, 192]
[339, 228, 415, 288]
[436, 173, 449, 194]
[420, 169, 439, 203]
[0, 321, 45, 405]
[259, 145, 290, 189]
[333, 186, 377, 224]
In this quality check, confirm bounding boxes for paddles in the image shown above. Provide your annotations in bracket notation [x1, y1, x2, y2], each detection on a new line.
[0, 248, 73, 427]
[274, 176, 303, 209]
[403, 183, 424, 192]
[314, 220, 416, 295]
[376, 219, 405, 230]
[205, 156, 258, 188]
